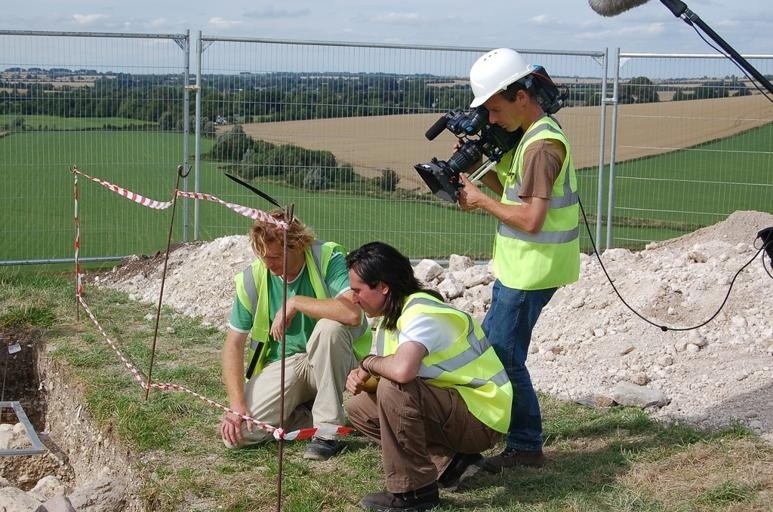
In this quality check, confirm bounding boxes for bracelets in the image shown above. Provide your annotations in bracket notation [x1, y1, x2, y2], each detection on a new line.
[367, 355, 382, 379]
[360, 353, 376, 372]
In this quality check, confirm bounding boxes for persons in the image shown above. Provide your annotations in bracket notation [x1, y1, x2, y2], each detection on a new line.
[219, 209, 370, 461]
[453, 46, 583, 472]
[340, 240, 515, 511]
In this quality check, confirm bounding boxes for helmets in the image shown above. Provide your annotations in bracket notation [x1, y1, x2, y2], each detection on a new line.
[468, 47, 539, 109]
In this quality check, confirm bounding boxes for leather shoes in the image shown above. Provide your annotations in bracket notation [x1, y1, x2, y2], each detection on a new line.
[301, 436, 340, 462]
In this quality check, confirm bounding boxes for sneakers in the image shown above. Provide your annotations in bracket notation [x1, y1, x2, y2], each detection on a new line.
[360, 483, 441, 512]
[484, 447, 548, 470]
